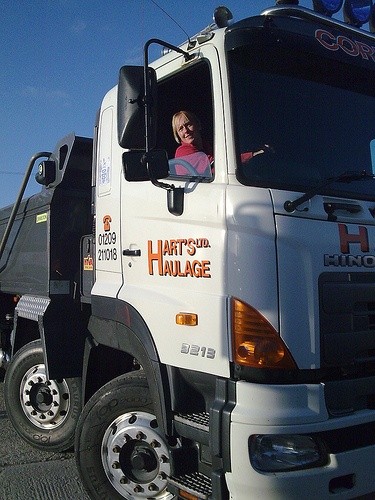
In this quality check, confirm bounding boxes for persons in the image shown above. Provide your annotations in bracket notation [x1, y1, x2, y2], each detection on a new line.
[169, 106, 276, 179]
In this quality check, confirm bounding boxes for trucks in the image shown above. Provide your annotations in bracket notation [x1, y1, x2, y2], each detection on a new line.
[0, 0, 375, 500]
